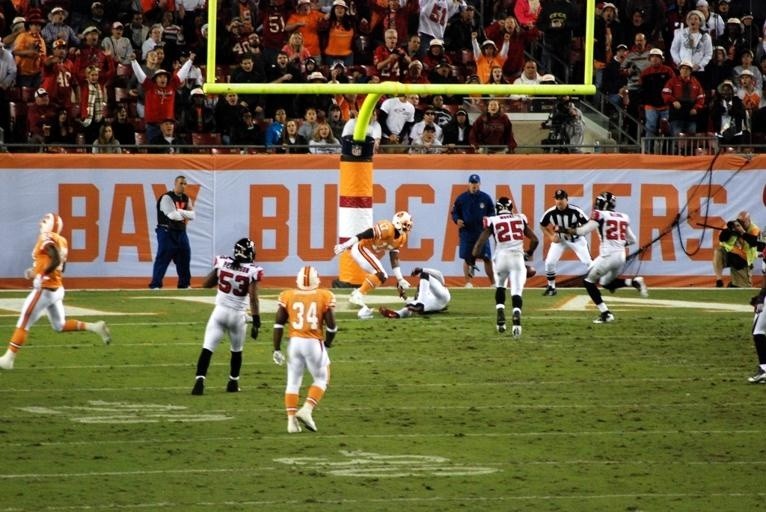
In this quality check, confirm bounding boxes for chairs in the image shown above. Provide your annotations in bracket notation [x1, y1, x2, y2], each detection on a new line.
[17, 44, 744, 156]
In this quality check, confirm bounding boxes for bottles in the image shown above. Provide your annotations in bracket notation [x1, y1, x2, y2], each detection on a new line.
[593, 139, 600, 153]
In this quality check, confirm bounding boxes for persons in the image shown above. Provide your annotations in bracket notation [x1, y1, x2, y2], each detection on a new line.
[380, 267, 451, 318]
[549, 191, 647, 324]
[191, 237, 265, 395]
[539, 189, 591, 296]
[713, 210, 760, 287]
[0, 0, 765, 154]
[272, 264, 338, 435]
[334, 212, 413, 308]
[746, 225, 766, 386]
[0, 213, 111, 371]
[149, 175, 197, 291]
[468, 196, 539, 338]
[452, 174, 496, 290]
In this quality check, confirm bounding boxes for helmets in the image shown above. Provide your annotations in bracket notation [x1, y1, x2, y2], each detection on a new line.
[392, 211, 412, 235]
[39, 213, 63, 235]
[296, 265, 320, 290]
[234, 238, 256, 263]
[496, 197, 513, 215]
[593, 192, 616, 210]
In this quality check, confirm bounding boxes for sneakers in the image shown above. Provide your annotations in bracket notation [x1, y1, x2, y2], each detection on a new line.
[0, 355, 14, 370]
[348, 293, 365, 307]
[192, 381, 203, 395]
[95, 320, 111, 345]
[286, 418, 301, 433]
[379, 306, 402, 318]
[542, 286, 556, 296]
[631, 276, 647, 297]
[295, 408, 317, 432]
[747, 365, 765, 384]
[495, 313, 505, 333]
[593, 311, 614, 324]
[512, 315, 521, 338]
[226, 381, 239, 392]
[405, 300, 424, 312]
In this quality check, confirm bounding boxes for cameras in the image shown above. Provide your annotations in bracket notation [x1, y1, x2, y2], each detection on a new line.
[727, 219, 744, 229]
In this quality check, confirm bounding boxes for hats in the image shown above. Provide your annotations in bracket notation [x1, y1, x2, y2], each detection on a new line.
[469, 175, 480, 183]
[601, 0, 754, 95]
[553, 190, 568, 200]
[11, 1, 166, 99]
[297, 1, 556, 84]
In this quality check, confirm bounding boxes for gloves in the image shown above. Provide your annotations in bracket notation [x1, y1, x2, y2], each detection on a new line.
[272, 350, 286, 366]
[333, 244, 345, 256]
[251, 325, 258, 339]
[398, 278, 411, 290]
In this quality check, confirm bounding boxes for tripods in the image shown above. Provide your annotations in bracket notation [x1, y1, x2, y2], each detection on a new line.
[541, 131, 572, 153]
